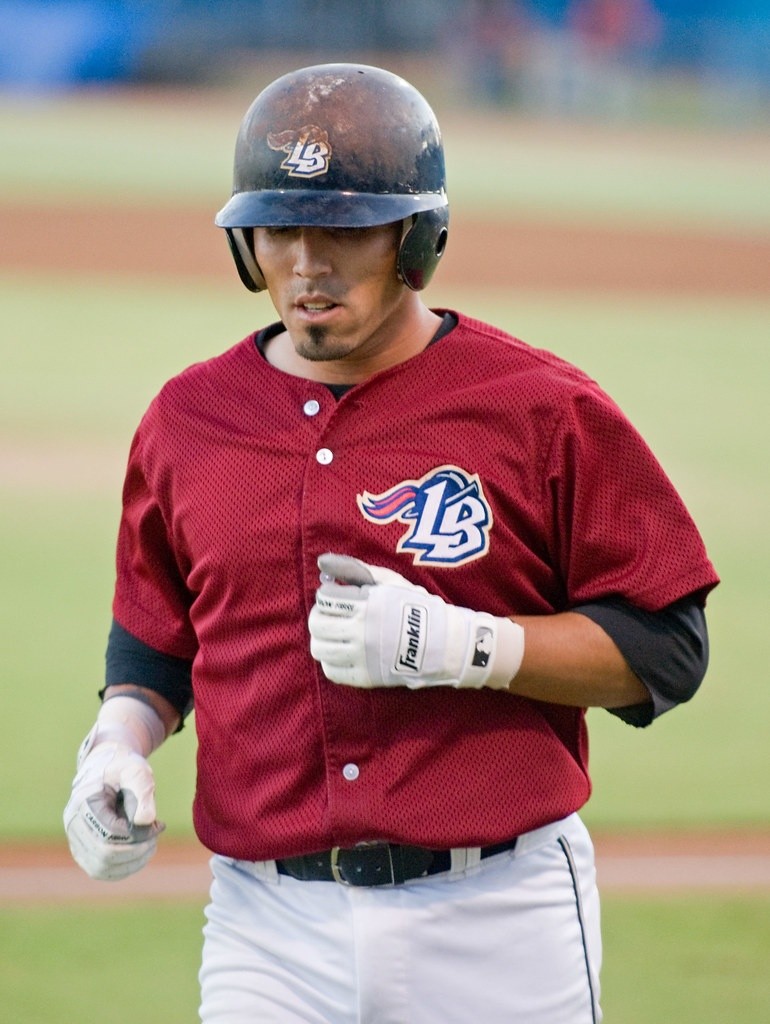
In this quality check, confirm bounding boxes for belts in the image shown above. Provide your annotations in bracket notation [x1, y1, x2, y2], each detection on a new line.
[277, 839, 520, 891]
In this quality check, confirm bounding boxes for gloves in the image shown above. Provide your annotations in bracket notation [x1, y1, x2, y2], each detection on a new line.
[65, 698, 170, 883]
[306, 551, 523, 695]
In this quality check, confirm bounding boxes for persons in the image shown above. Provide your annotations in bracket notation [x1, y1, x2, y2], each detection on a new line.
[60, 60, 722, 1024]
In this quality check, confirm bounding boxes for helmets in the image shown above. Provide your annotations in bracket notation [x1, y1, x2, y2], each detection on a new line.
[210, 62, 460, 292]
